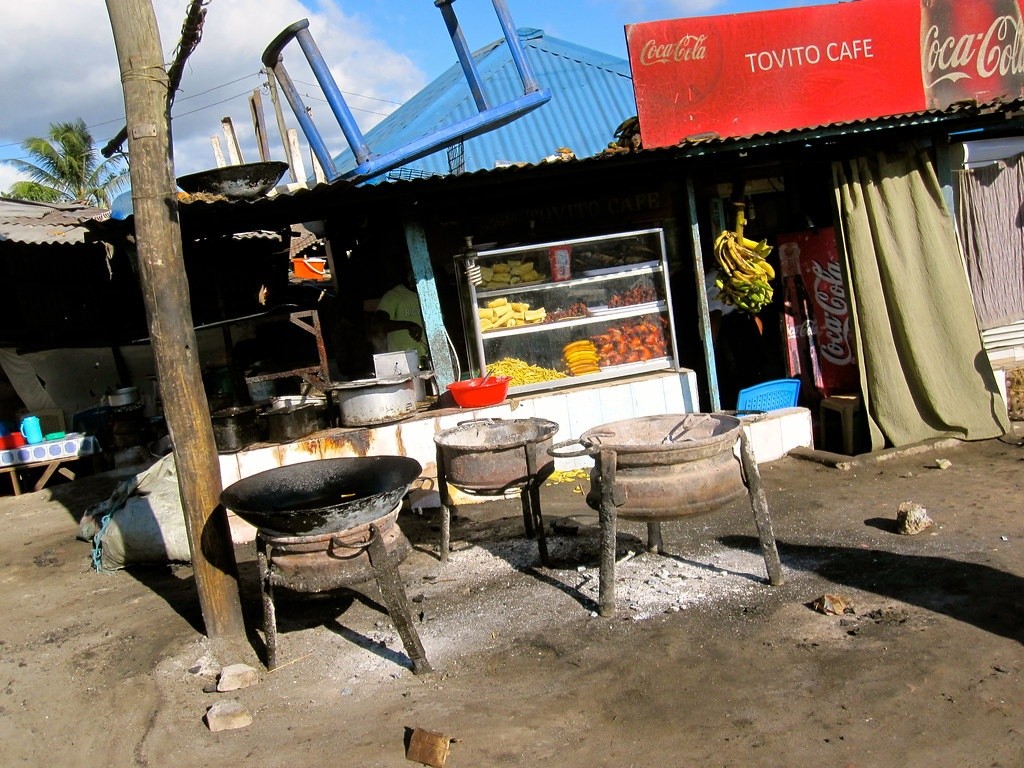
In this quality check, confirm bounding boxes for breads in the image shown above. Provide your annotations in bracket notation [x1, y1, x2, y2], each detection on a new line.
[477, 261, 549, 333]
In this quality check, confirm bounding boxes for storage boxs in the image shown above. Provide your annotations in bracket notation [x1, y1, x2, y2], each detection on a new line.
[731, 405, 812, 466]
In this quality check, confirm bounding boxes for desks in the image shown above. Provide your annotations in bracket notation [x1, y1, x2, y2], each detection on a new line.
[0, 456, 81, 496]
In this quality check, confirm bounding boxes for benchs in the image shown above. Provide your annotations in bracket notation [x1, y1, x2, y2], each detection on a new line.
[819, 391, 863, 457]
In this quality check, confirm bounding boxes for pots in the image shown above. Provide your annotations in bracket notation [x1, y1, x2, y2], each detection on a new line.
[211, 403, 259, 453]
[336, 379, 417, 426]
[174, 160, 291, 201]
[218, 455, 423, 538]
[269, 402, 318, 445]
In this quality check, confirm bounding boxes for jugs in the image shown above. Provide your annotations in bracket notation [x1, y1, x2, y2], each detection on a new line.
[20, 415, 43, 444]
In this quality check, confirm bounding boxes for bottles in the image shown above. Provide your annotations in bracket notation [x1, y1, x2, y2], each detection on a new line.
[918, 0, 1024, 111]
[777, 239, 819, 408]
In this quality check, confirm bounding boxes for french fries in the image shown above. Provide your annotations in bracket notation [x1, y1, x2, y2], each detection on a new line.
[485, 358, 566, 386]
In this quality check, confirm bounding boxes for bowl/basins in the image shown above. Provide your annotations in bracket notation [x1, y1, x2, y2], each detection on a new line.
[0, 432, 26, 450]
[445, 376, 514, 408]
[108, 385, 141, 406]
[434, 418, 559, 497]
[45, 431, 65, 441]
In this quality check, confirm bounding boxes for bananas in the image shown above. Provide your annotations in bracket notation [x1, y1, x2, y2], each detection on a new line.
[711, 232, 775, 313]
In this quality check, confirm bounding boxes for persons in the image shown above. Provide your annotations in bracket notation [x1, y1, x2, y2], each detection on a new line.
[371, 262, 431, 372]
[706, 258, 759, 411]
